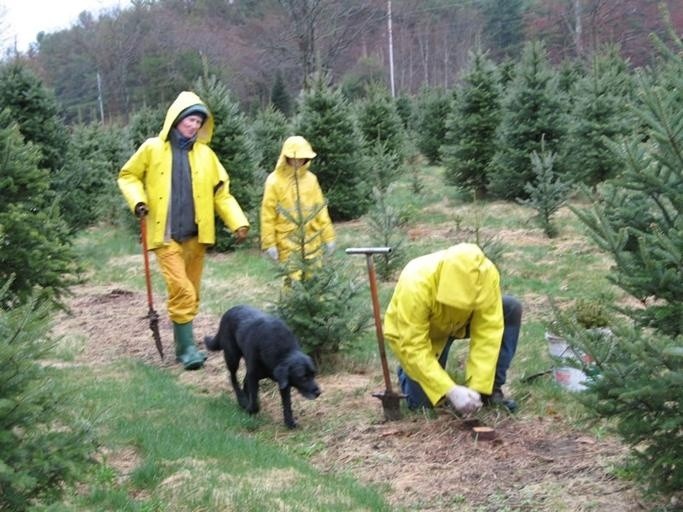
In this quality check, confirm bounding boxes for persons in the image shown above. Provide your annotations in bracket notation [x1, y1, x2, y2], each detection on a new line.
[113, 90, 251, 372]
[379, 242, 520, 414]
[259, 133, 337, 319]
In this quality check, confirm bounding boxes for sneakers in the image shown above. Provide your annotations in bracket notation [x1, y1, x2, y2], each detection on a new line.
[481, 388, 518, 413]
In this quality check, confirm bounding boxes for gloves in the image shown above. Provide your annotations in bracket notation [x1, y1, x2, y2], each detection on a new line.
[326, 240, 335, 253]
[266, 247, 279, 261]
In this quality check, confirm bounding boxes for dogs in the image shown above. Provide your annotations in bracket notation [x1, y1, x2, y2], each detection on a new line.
[203, 304, 323, 430]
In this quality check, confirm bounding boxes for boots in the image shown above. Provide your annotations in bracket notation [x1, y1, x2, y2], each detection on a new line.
[172, 320, 209, 369]
